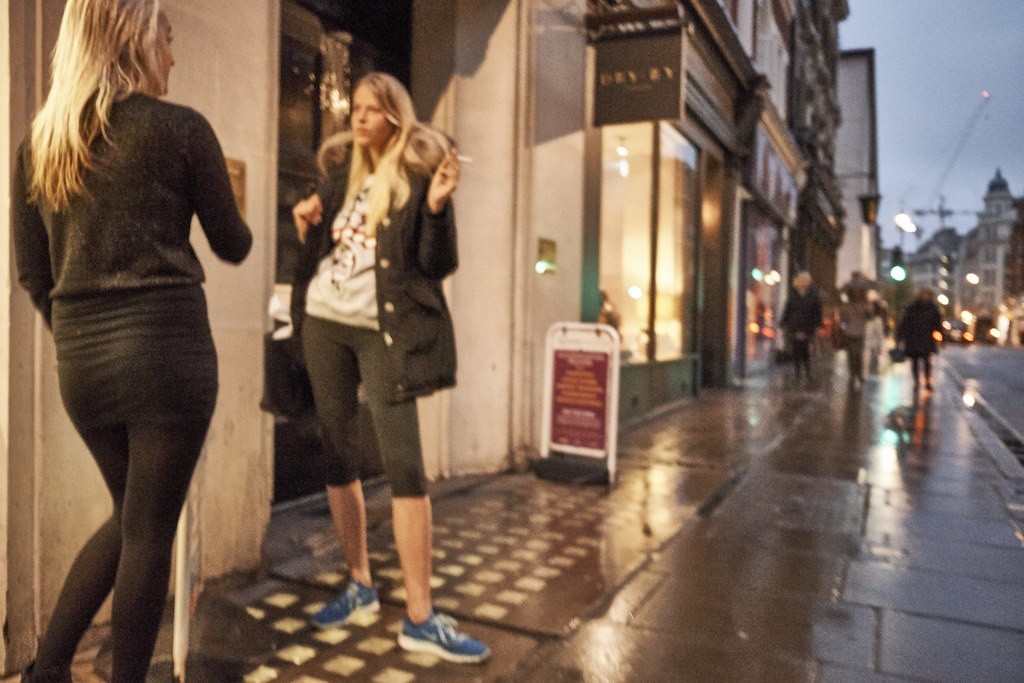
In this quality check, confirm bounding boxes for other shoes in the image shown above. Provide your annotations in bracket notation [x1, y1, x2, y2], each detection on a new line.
[911, 383, 936, 391]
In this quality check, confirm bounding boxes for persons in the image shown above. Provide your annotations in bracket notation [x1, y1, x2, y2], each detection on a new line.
[289, 72, 495, 665]
[13, 0, 252, 682]
[778, 268, 824, 382]
[889, 290, 944, 390]
[834, 290, 881, 382]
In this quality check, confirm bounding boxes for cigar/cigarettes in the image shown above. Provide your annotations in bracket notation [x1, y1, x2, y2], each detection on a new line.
[452, 153, 474, 162]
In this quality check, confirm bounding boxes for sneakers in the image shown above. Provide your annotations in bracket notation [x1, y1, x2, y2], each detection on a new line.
[396, 611, 492, 666]
[307, 576, 380, 628]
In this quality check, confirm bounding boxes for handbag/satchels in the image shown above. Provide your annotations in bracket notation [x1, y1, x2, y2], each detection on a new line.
[890, 348, 907, 362]
[830, 321, 846, 349]
